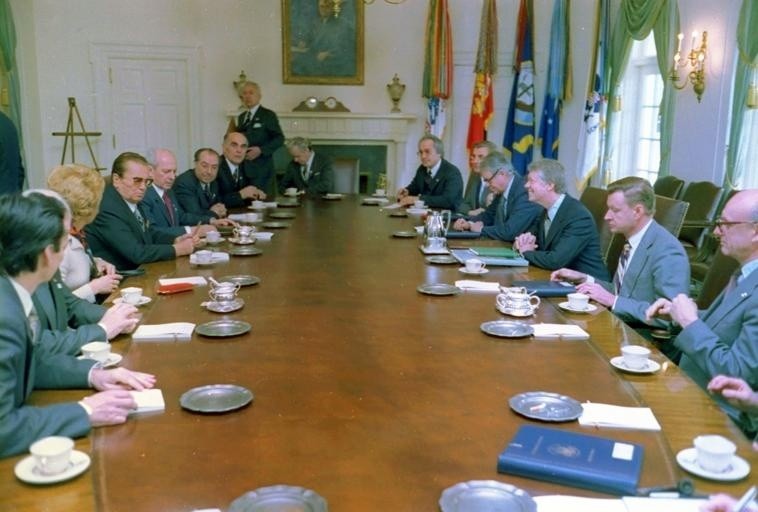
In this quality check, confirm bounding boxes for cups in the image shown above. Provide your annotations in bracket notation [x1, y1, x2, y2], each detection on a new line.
[375, 189, 386, 194]
[118, 287, 145, 303]
[494, 283, 538, 319]
[80, 340, 113, 364]
[567, 293, 591, 311]
[206, 279, 240, 306]
[234, 225, 252, 240]
[620, 343, 649, 368]
[252, 199, 263, 206]
[413, 200, 425, 209]
[29, 437, 75, 472]
[694, 433, 737, 471]
[286, 187, 298, 193]
[464, 258, 486, 272]
[193, 230, 220, 260]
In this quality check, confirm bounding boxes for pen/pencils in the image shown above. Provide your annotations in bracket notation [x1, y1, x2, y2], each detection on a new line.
[733, 486, 758, 512]
[191, 219, 202, 238]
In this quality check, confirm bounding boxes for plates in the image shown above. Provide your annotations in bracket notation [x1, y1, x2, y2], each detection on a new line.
[322, 195, 343, 200]
[16, 451, 95, 487]
[675, 448, 752, 482]
[180, 381, 254, 412]
[74, 351, 123, 369]
[390, 213, 407, 216]
[392, 231, 417, 239]
[361, 200, 381, 205]
[425, 254, 458, 264]
[244, 204, 264, 226]
[187, 256, 221, 267]
[219, 274, 261, 288]
[559, 302, 598, 314]
[226, 234, 260, 259]
[609, 356, 661, 373]
[407, 209, 430, 214]
[371, 193, 389, 197]
[263, 201, 302, 228]
[459, 266, 490, 275]
[417, 282, 460, 297]
[196, 320, 253, 338]
[510, 389, 586, 421]
[112, 294, 156, 308]
[206, 300, 249, 313]
[481, 320, 536, 338]
[223, 484, 328, 512]
[437, 476, 537, 512]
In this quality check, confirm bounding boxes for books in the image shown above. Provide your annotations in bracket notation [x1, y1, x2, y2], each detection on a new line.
[439, 227, 481, 240]
[526, 322, 589, 341]
[509, 279, 577, 296]
[494, 422, 644, 500]
[467, 245, 519, 258]
[447, 248, 530, 267]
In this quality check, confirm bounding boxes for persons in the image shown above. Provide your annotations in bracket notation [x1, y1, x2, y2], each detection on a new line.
[455, 140, 497, 216]
[642, 185, 757, 444]
[278, 136, 336, 197]
[394, 133, 463, 212]
[549, 175, 692, 354]
[289, 0, 354, 77]
[511, 158, 611, 282]
[696, 492, 757, 512]
[703, 371, 757, 455]
[0, 111, 25, 195]
[236, 81, 284, 202]
[450, 151, 546, 251]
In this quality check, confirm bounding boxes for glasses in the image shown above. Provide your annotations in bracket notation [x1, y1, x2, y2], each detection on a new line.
[417, 151, 433, 156]
[713, 216, 753, 231]
[123, 176, 153, 187]
[483, 169, 500, 183]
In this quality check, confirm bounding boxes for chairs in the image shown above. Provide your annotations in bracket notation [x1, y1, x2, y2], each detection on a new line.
[326, 156, 361, 194]
[576, 170, 751, 328]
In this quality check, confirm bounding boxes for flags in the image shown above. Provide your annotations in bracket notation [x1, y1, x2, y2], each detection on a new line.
[502, 1, 536, 176]
[420, 0, 455, 140]
[464, 1, 500, 171]
[535, 0, 574, 158]
[572, 0, 623, 191]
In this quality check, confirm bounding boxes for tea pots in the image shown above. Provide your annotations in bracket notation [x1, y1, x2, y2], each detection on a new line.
[419, 209, 450, 252]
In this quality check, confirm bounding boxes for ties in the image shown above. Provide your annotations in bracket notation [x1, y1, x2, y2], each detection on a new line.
[233, 169, 238, 182]
[135, 209, 146, 232]
[303, 165, 308, 180]
[615, 241, 632, 294]
[243, 111, 251, 129]
[29, 310, 41, 344]
[205, 183, 211, 198]
[721, 267, 743, 304]
[426, 170, 432, 187]
[498, 196, 506, 223]
[544, 213, 551, 241]
[163, 192, 175, 224]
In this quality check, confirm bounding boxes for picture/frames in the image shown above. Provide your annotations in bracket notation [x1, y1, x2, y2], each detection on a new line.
[279, 0, 365, 86]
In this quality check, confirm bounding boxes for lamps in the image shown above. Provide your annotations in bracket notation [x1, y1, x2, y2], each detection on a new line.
[671, 27, 708, 102]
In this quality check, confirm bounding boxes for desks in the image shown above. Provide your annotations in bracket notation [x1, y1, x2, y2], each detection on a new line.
[1, 186, 757, 512]
